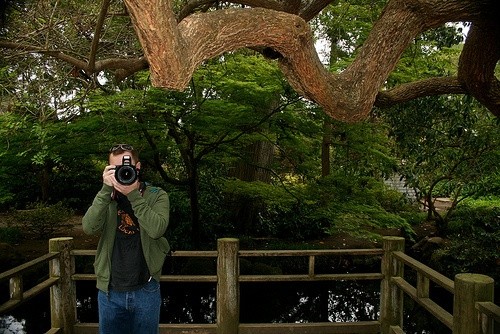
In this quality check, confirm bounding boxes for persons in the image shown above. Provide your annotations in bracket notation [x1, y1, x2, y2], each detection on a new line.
[82, 143, 169, 334]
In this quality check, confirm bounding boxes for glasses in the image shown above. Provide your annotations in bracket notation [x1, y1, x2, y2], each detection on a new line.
[109, 144, 135, 152]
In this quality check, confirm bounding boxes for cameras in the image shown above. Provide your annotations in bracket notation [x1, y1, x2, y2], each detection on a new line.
[114, 155, 139, 185]
